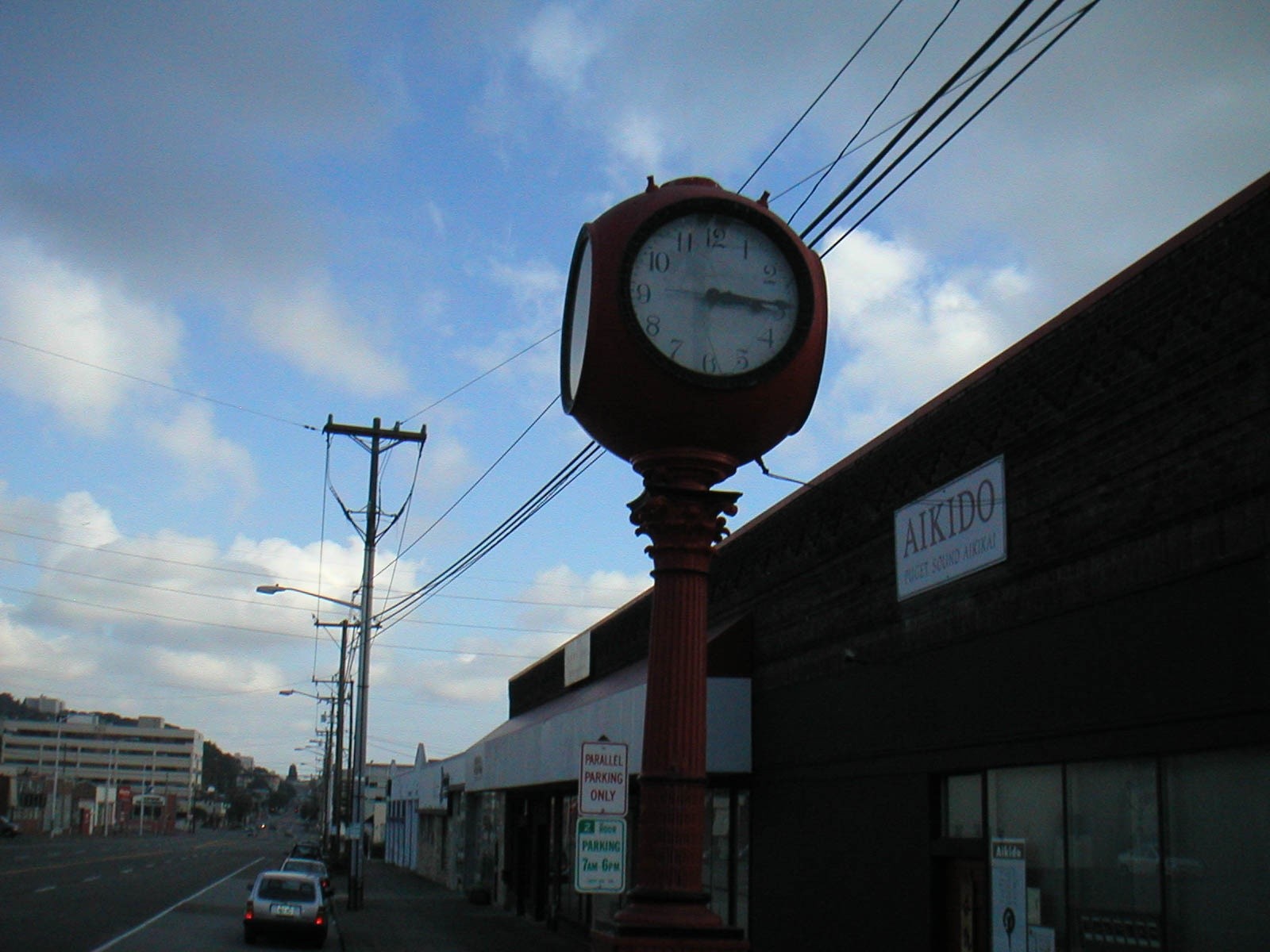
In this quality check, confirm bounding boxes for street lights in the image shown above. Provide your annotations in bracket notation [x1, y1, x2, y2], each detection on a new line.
[255, 584, 362, 910]
[279, 688, 337, 851]
[50, 713, 83, 838]
[103, 736, 140, 837]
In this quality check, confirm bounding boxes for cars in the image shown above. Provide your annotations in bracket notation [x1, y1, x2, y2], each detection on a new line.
[243, 872, 328, 950]
[0, 815, 20, 836]
[279, 839, 334, 897]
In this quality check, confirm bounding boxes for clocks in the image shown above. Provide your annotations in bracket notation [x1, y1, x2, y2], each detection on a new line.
[630, 208, 801, 375]
